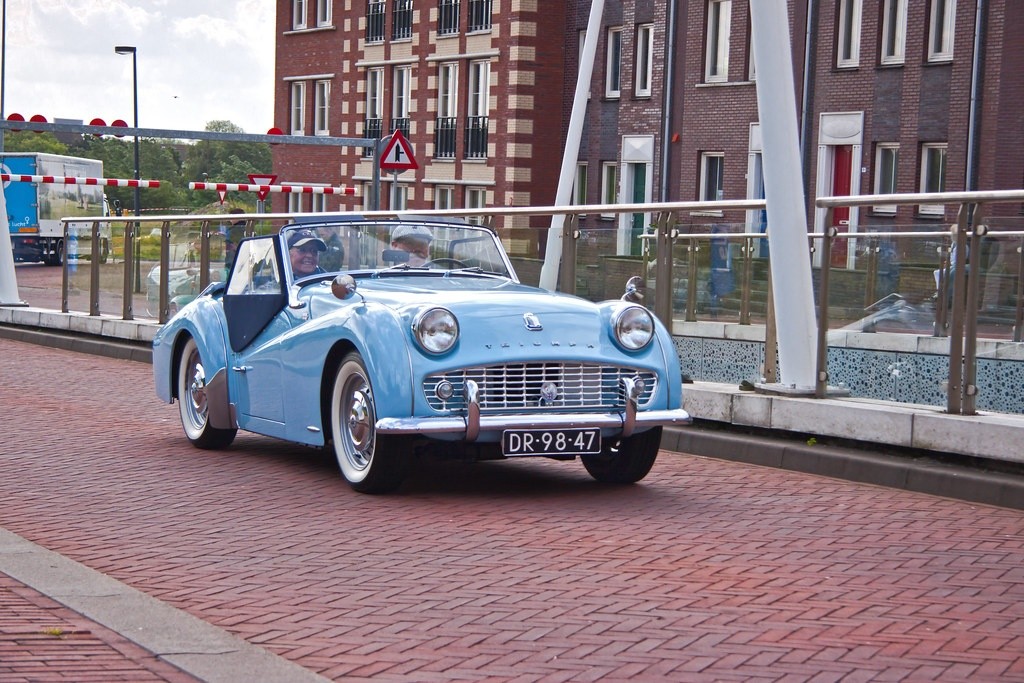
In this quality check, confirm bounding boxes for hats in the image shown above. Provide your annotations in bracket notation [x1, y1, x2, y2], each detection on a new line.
[287, 231, 327, 253]
[391, 224, 433, 241]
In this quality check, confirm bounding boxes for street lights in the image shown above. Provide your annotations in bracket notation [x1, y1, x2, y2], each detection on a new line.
[113, 45, 144, 297]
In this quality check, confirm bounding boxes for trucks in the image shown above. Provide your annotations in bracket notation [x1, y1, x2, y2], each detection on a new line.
[0, 150, 124, 268]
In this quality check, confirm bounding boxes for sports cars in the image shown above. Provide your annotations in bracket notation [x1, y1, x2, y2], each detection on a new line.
[156, 219, 694, 496]
[145, 232, 250, 323]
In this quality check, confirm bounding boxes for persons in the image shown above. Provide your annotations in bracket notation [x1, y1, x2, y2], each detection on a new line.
[931, 224, 969, 298]
[391, 224, 433, 270]
[224, 209, 258, 270]
[318, 226, 344, 272]
[256, 229, 328, 290]
[83, 193, 88, 210]
